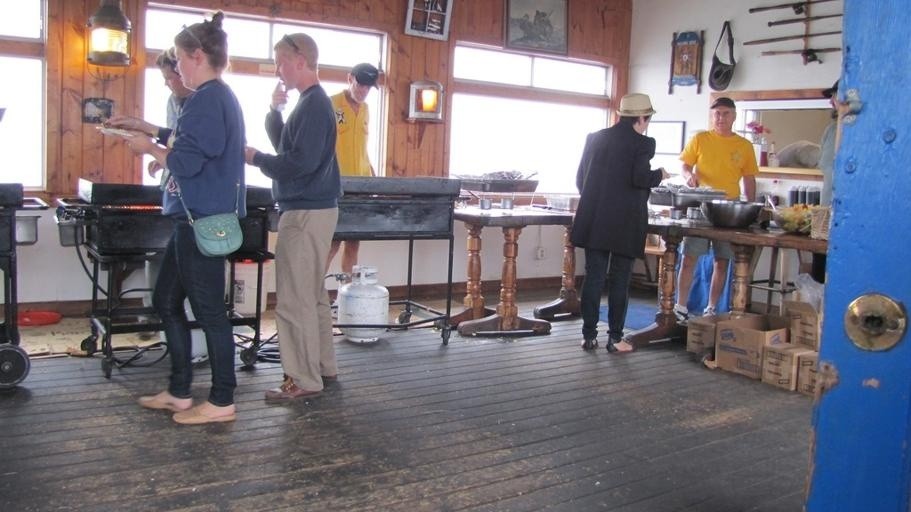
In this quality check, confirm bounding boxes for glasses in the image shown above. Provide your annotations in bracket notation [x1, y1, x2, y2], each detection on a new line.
[181, 23, 202, 49]
[282, 34, 299, 49]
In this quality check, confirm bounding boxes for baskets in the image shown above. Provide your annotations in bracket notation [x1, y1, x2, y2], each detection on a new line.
[809, 206, 831, 239]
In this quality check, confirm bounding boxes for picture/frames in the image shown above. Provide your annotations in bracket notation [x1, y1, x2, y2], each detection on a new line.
[503, 0, 572, 59]
[403, 0, 454, 41]
[646, 120, 685, 155]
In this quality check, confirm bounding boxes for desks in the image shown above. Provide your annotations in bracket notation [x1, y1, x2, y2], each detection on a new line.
[455, 207, 572, 334]
[534, 211, 828, 350]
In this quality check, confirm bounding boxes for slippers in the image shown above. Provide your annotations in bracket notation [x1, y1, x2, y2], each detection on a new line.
[172, 407, 236, 424]
[138, 395, 187, 412]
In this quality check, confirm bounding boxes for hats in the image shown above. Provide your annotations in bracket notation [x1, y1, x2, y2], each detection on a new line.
[351, 63, 379, 90]
[821, 80, 838, 97]
[616, 93, 656, 117]
[710, 98, 735, 108]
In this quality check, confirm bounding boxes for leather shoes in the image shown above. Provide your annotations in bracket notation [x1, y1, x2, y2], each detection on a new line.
[265, 376, 321, 401]
[283, 374, 338, 382]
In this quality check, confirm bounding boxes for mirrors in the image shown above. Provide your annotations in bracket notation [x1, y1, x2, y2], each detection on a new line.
[708, 89, 831, 183]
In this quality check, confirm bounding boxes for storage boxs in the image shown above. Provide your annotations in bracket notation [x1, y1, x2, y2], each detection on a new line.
[687, 302, 822, 400]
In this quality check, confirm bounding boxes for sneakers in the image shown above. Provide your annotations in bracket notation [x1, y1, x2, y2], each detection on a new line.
[330, 299, 338, 318]
[703, 306, 716, 317]
[673, 309, 694, 324]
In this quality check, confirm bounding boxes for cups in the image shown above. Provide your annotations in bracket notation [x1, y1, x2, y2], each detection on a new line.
[500, 197, 513, 210]
[479, 198, 492, 210]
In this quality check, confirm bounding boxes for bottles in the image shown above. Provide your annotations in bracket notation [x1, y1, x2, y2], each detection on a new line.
[769, 180, 780, 209]
[786, 184, 821, 208]
[760, 137, 780, 168]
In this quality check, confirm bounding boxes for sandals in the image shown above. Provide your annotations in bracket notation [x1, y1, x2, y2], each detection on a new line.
[606, 340, 637, 352]
[581, 338, 598, 349]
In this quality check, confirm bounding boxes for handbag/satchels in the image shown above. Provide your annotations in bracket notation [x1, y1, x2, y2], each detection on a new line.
[708, 21, 735, 91]
[192, 211, 244, 257]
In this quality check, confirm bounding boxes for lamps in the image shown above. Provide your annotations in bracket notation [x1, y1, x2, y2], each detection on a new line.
[83, 0, 132, 65]
[407, 80, 443, 119]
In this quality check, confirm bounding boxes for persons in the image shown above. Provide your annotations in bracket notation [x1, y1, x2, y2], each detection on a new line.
[568, 93, 671, 354]
[143, 44, 209, 365]
[672, 97, 760, 315]
[106, 10, 248, 425]
[244, 32, 344, 402]
[318, 62, 380, 286]
[811, 78, 840, 284]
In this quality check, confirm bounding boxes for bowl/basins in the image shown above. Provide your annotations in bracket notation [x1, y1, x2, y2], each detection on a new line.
[696, 198, 766, 228]
